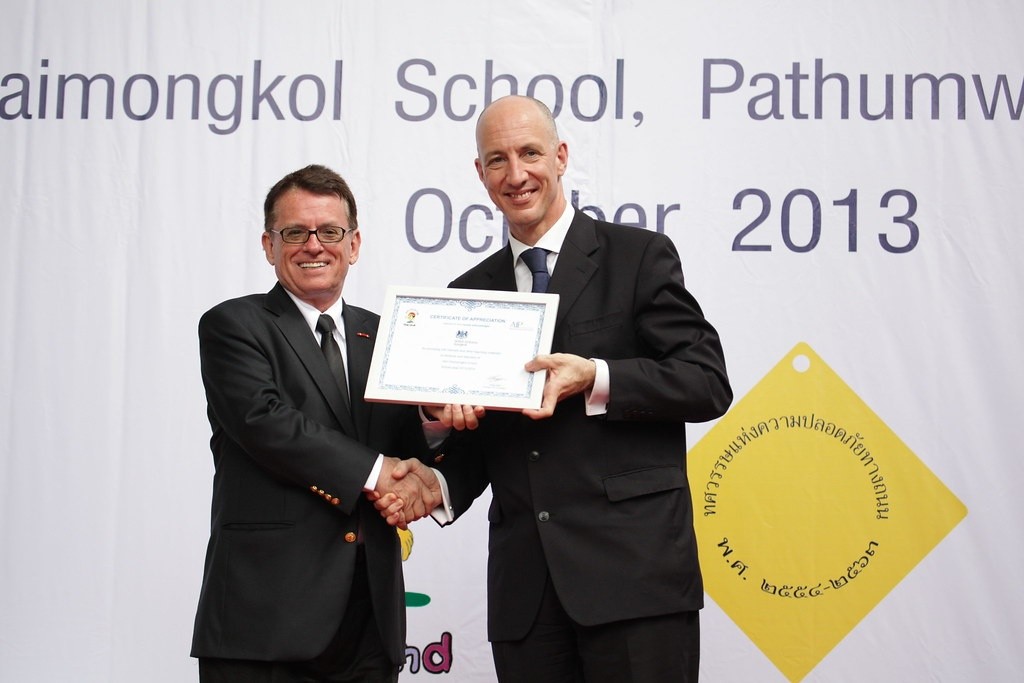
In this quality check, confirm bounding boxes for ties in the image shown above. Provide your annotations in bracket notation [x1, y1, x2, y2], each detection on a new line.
[520, 248, 553, 294]
[316, 314, 351, 420]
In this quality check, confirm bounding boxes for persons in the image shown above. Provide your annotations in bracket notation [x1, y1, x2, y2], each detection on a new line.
[190, 164, 487, 683]
[368, 96, 734, 683]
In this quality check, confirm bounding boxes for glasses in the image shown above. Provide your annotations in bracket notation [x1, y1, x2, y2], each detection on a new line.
[271, 226, 352, 244]
